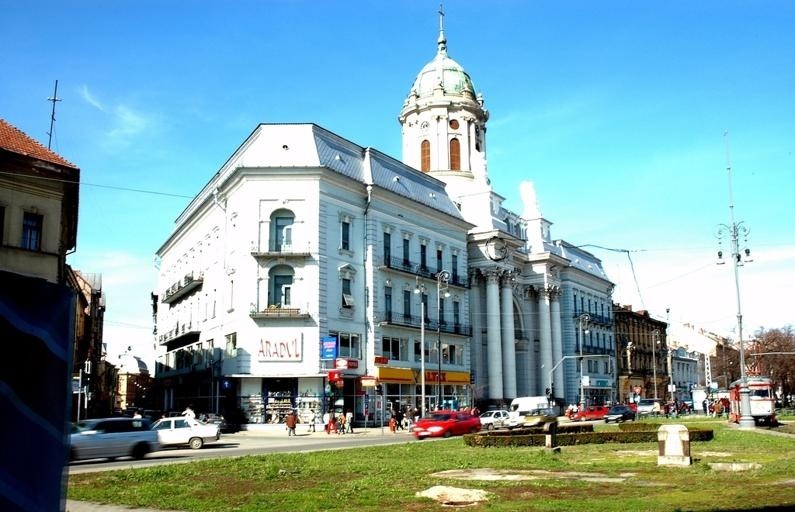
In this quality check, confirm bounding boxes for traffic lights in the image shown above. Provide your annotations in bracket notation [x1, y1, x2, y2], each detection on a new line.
[79, 368, 89, 388]
[545, 388, 550, 395]
[375, 384, 382, 394]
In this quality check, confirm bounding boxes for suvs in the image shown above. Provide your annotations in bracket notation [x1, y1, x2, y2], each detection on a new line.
[65, 417, 158, 462]
[635, 398, 664, 417]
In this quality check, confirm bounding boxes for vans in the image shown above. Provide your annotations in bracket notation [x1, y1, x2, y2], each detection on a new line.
[507, 395, 551, 417]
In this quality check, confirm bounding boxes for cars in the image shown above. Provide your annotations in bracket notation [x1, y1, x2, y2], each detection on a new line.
[683, 399, 693, 408]
[653, 401, 688, 415]
[413, 410, 482, 438]
[148, 417, 222, 452]
[703, 397, 718, 414]
[196, 411, 226, 430]
[571, 404, 608, 421]
[522, 408, 557, 428]
[502, 410, 532, 428]
[115, 405, 182, 419]
[478, 409, 511, 430]
[603, 404, 636, 423]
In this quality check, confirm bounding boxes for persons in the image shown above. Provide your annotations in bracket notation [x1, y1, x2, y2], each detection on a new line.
[323, 409, 353, 435]
[133, 410, 143, 428]
[459, 405, 481, 416]
[702, 395, 730, 417]
[389, 399, 450, 434]
[567, 402, 582, 417]
[604, 398, 626, 409]
[285, 410, 297, 436]
[308, 409, 316, 432]
[182, 404, 196, 418]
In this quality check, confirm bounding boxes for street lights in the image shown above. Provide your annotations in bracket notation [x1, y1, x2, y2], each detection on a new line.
[625, 341, 637, 374]
[413, 264, 429, 419]
[435, 269, 452, 409]
[715, 217, 759, 428]
[579, 313, 591, 405]
[651, 329, 663, 398]
